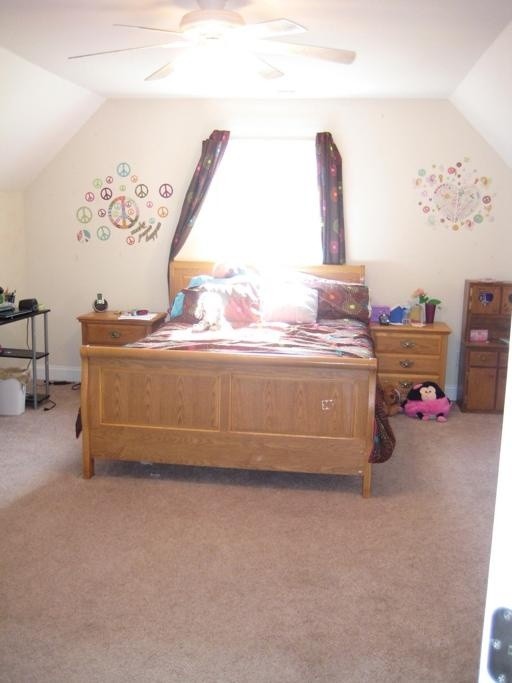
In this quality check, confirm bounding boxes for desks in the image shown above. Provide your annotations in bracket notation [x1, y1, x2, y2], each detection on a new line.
[1, 302, 52, 415]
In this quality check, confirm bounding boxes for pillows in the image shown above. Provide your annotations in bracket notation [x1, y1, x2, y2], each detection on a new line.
[179, 268, 372, 332]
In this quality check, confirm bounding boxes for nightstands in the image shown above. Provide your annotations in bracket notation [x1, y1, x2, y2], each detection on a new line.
[77, 308, 168, 349]
[369, 320, 453, 404]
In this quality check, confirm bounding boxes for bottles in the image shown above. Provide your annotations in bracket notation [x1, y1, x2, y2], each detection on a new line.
[379, 311, 390, 327]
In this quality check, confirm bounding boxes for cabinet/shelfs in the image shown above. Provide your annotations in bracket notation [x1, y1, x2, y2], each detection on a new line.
[456, 280, 512, 419]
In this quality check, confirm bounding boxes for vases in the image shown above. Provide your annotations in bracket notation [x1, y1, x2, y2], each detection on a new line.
[425, 306, 435, 323]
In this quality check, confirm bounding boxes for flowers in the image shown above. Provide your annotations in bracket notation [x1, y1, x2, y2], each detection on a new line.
[415, 287, 440, 306]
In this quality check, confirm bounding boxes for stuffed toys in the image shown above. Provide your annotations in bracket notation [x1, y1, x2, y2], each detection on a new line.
[382, 383, 402, 416]
[404, 381, 452, 421]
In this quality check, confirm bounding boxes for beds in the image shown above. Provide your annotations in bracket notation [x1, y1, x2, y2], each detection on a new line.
[78, 261, 379, 503]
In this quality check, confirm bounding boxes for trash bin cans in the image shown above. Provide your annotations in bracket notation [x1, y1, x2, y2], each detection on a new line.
[1, 368, 29, 416]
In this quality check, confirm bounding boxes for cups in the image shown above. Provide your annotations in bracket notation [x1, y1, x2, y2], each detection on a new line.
[402, 311, 411, 325]
